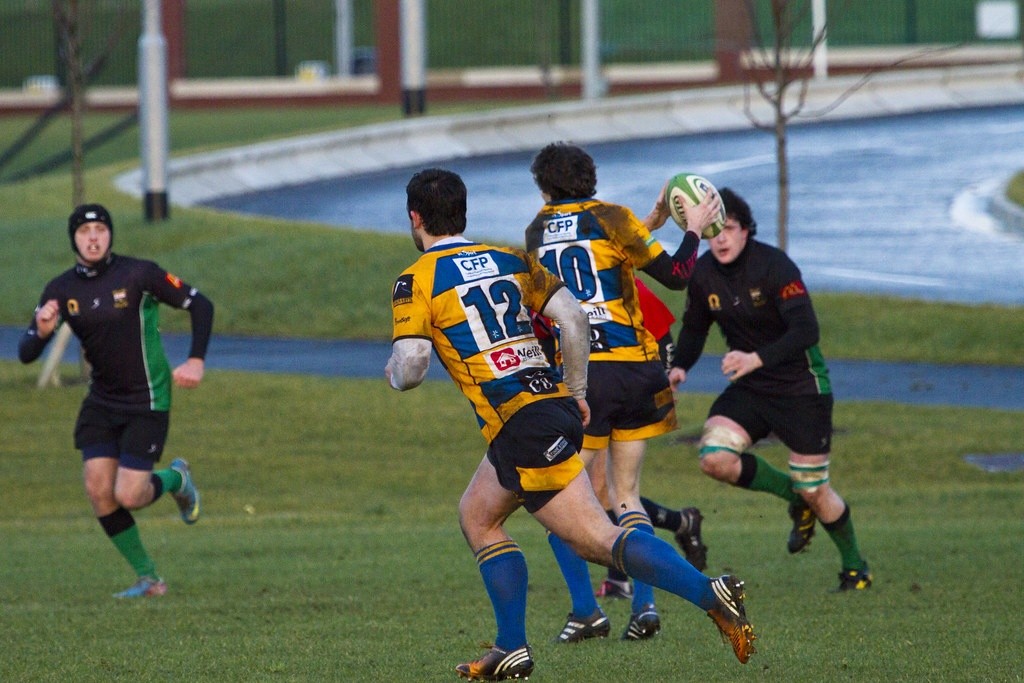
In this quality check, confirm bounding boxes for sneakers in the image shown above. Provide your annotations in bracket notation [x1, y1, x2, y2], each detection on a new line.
[622, 608, 661, 640]
[595, 577, 633, 601]
[168, 455, 201, 525]
[113, 578, 168, 598]
[455, 643, 535, 683]
[706, 575, 758, 665]
[787, 501, 816, 554]
[674, 507, 708, 572]
[830, 561, 873, 593]
[551, 608, 611, 644]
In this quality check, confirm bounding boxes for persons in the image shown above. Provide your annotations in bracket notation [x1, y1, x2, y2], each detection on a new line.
[664, 187, 874, 591]
[522, 142, 720, 642]
[18, 203, 215, 602]
[384, 167, 758, 683]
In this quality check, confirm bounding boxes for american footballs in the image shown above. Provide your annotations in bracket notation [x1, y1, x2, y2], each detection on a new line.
[665, 171, 727, 239]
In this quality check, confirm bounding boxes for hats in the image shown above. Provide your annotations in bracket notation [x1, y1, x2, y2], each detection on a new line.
[69, 204, 112, 250]
